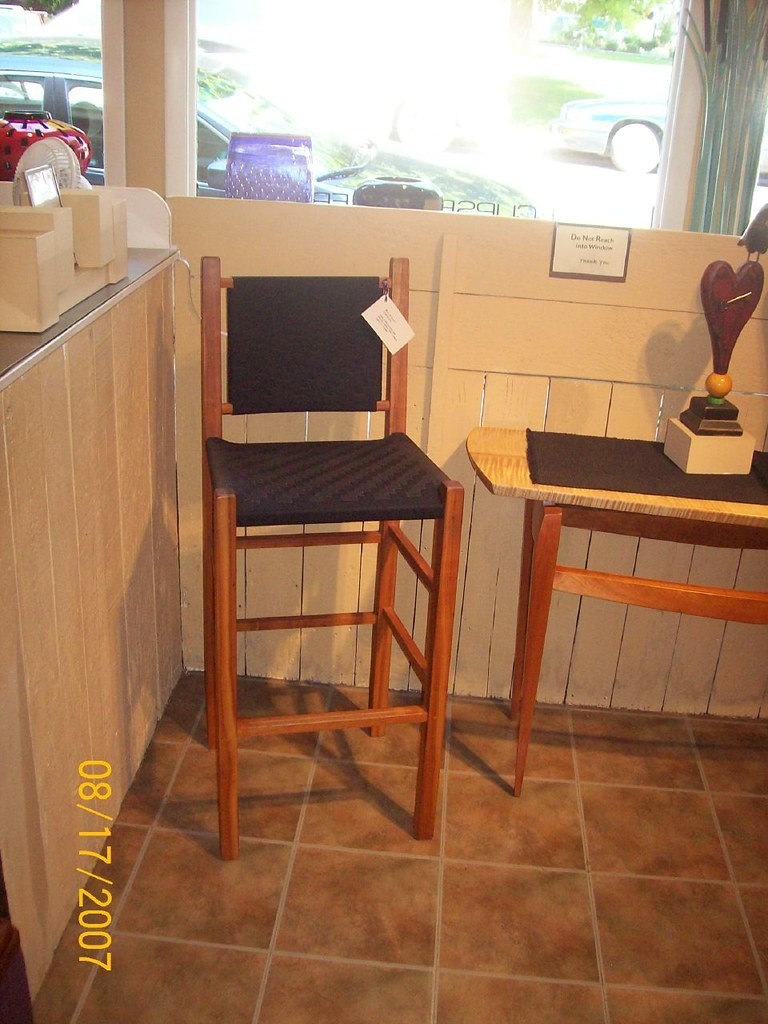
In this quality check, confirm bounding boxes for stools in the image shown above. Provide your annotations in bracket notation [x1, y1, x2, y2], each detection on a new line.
[200, 253, 466, 859]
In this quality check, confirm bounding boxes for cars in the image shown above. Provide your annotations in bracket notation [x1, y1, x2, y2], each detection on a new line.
[556, 62, 768, 186]
[0, 36, 538, 220]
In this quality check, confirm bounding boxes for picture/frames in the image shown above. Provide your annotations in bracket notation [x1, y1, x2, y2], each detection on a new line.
[24, 164, 62, 208]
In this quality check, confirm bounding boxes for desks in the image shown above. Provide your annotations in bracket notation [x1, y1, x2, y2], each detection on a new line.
[468, 429, 768, 796]
